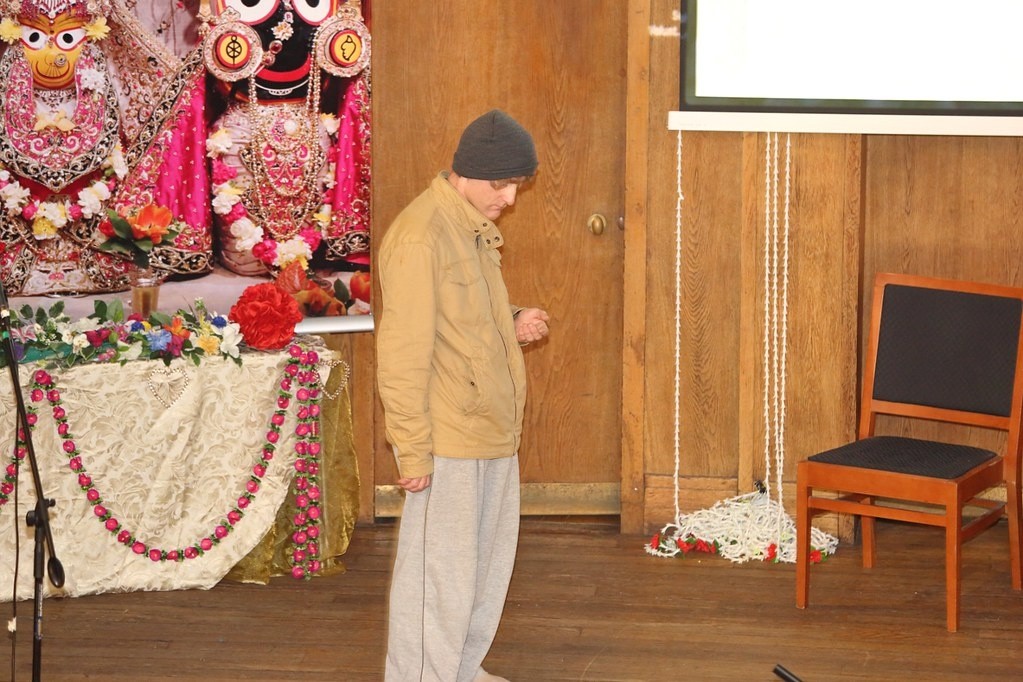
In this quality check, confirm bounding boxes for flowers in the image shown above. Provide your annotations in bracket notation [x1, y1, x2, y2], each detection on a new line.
[0, 14, 342, 576]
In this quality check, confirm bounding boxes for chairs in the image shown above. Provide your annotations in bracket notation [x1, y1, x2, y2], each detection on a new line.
[795, 273, 1019, 633]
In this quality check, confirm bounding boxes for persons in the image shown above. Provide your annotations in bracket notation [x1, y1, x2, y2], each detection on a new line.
[376, 106, 551, 682]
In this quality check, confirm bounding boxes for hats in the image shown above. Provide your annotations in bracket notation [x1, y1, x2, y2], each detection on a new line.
[452, 109, 537, 180]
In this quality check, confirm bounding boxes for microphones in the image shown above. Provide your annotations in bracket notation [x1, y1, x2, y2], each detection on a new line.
[0, 280, 11, 326]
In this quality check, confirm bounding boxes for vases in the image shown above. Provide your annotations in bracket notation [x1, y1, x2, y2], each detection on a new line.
[131, 263, 159, 319]
